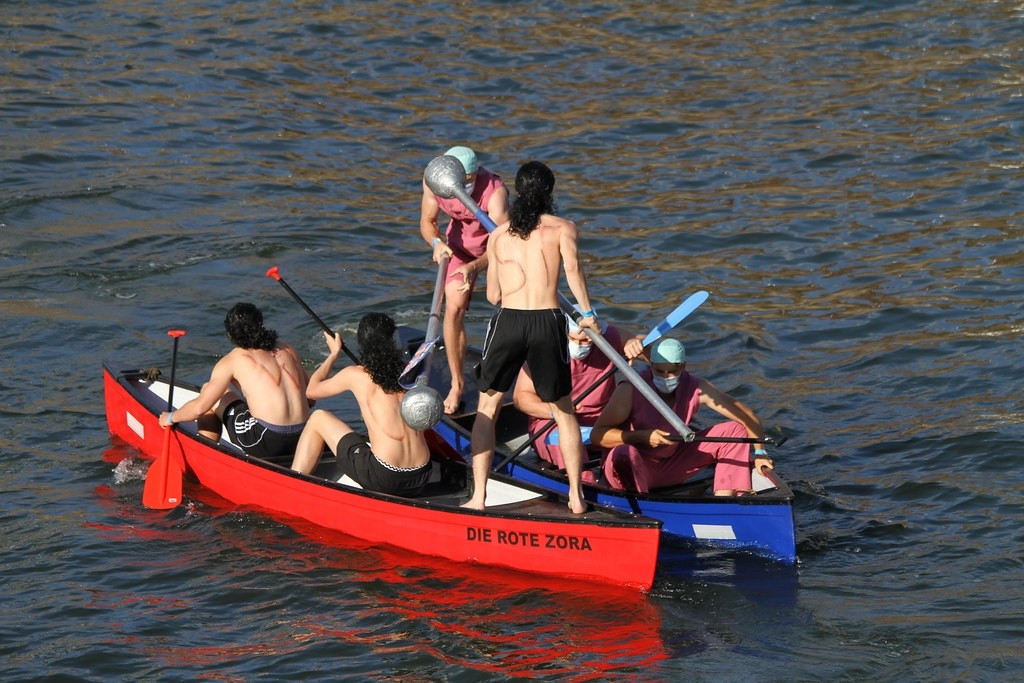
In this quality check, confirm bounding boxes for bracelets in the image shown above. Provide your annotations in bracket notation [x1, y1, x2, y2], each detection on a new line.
[581, 306, 599, 320]
[168, 412, 173, 423]
[755, 449, 768, 456]
[432, 238, 440, 250]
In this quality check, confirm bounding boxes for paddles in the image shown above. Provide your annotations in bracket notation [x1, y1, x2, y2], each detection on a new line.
[493, 290, 709, 473]
[543, 426, 789, 447]
[142, 329, 187, 510]
[266, 266, 362, 365]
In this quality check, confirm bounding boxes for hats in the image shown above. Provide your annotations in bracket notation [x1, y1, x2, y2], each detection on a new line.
[565, 303, 598, 333]
[648, 336, 685, 363]
[443, 145, 479, 174]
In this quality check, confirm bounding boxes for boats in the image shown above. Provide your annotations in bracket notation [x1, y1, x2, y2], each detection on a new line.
[102, 359, 664, 591]
[398, 325, 796, 563]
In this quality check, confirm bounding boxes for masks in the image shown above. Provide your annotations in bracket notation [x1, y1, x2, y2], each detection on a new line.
[464, 181, 476, 195]
[569, 339, 592, 360]
[652, 369, 682, 394]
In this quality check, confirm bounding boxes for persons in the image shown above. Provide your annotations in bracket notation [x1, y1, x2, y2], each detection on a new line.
[590, 339, 773, 497]
[291, 314, 434, 490]
[512, 303, 648, 483]
[421, 147, 510, 414]
[159, 303, 316, 457]
[458, 161, 603, 514]
